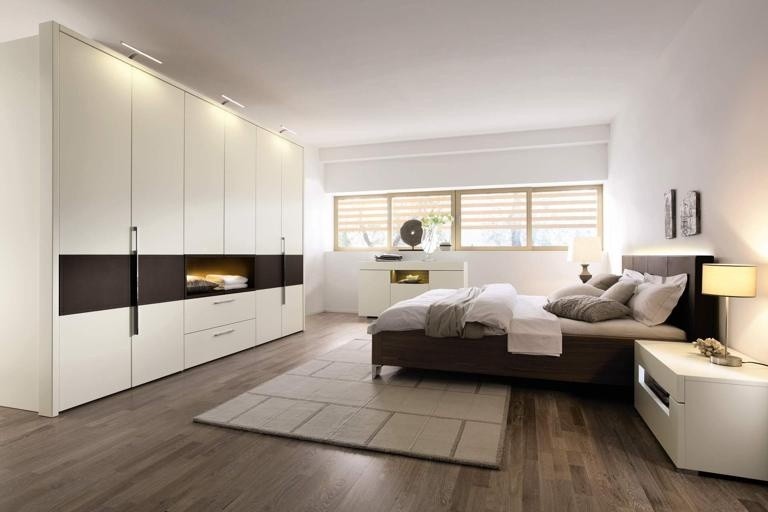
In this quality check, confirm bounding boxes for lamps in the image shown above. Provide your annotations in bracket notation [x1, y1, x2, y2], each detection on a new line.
[700, 261, 758, 369]
[569, 237, 600, 284]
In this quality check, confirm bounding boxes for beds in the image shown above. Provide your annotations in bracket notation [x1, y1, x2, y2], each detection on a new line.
[367, 254, 720, 386]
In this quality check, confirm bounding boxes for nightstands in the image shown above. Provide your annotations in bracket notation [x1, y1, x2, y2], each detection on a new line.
[634, 339, 768, 482]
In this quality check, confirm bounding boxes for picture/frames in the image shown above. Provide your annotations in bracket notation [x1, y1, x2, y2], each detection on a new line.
[663, 188, 676, 239]
[680, 190, 702, 237]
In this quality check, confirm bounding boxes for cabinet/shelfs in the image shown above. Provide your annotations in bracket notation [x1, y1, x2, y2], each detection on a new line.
[356, 260, 390, 321]
[390, 260, 430, 308]
[430, 260, 469, 290]
[256, 123, 308, 346]
[183, 84, 257, 373]
[0, 20, 185, 417]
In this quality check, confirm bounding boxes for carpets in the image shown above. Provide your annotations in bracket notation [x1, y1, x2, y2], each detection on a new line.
[192, 337, 511, 471]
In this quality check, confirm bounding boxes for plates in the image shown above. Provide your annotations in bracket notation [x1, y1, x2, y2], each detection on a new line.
[188, 279, 218, 292]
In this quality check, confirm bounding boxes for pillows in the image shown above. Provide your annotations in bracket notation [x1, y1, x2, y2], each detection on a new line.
[617, 268, 645, 283]
[626, 272, 688, 328]
[585, 273, 622, 291]
[543, 294, 634, 324]
[547, 284, 605, 304]
[599, 268, 644, 304]
[186, 276, 217, 294]
